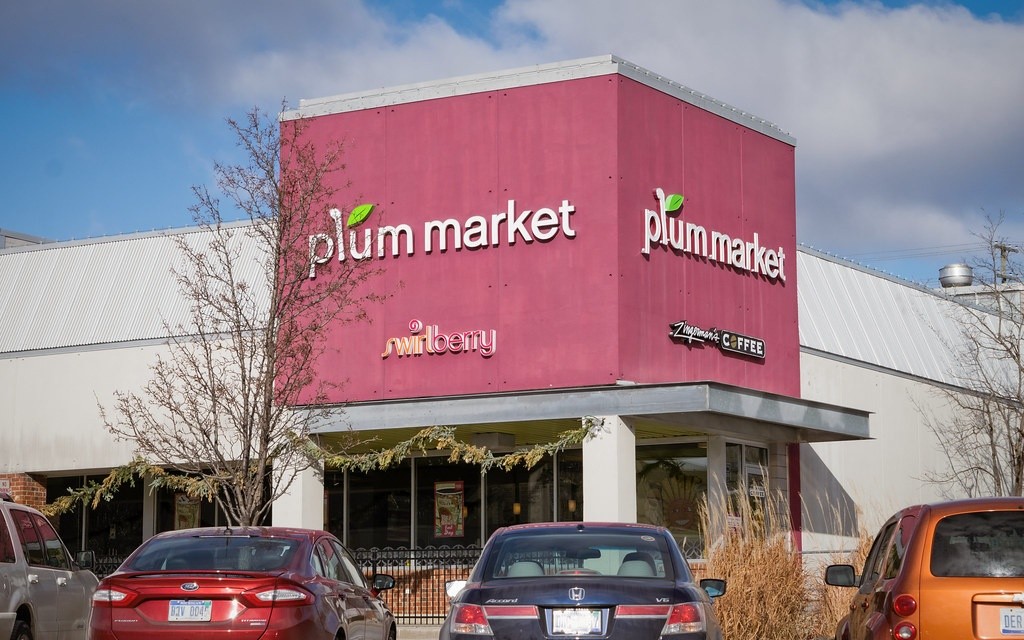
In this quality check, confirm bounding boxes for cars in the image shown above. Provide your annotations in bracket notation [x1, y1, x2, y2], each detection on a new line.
[440, 520, 726, 640]
[86, 524, 398, 640]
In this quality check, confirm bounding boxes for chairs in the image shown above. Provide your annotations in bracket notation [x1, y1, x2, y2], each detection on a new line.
[617, 553, 658, 577]
[507, 562, 545, 577]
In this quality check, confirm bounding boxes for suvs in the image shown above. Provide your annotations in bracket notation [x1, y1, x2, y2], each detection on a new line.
[825, 497, 1024, 640]
[0, 492, 102, 639]
